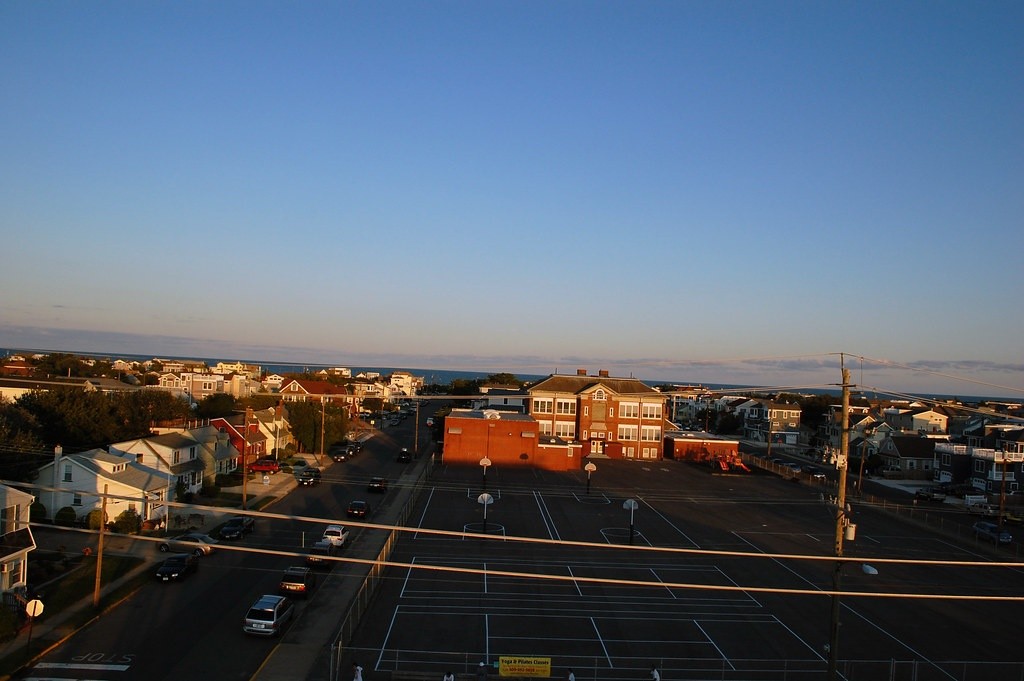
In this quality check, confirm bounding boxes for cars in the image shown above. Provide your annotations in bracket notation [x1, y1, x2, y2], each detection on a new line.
[306, 541, 337, 566]
[288, 459, 311, 475]
[915, 485, 946, 502]
[358, 397, 417, 426]
[246, 457, 279, 474]
[968, 500, 1024, 524]
[348, 500, 370, 520]
[323, 525, 350, 549]
[218, 516, 255, 541]
[241, 594, 295, 639]
[157, 531, 219, 558]
[748, 451, 826, 481]
[397, 450, 412, 464]
[333, 440, 363, 464]
[155, 552, 198, 582]
[368, 477, 386, 492]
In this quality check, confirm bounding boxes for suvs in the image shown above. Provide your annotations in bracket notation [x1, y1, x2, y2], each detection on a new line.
[298, 467, 323, 487]
[972, 522, 1012, 546]
[280, 565, 315, 599]
